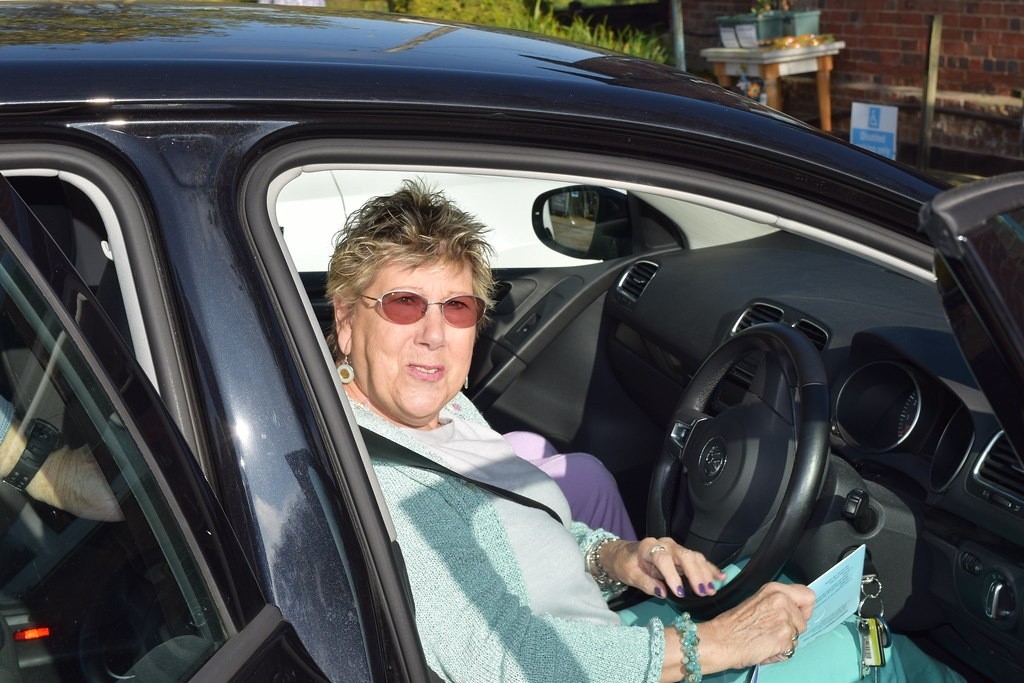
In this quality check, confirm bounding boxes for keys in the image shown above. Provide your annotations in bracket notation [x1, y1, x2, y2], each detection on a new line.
[860, 617, 886, 683]
[861, 573, 877, 584]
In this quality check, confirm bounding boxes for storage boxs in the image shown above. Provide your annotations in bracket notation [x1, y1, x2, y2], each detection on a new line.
[715, 9, 820, 48]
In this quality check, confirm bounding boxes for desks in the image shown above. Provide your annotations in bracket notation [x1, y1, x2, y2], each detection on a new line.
[700, 40, 848, 133]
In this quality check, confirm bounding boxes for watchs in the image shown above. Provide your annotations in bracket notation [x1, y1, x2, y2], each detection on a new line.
[2, 417, 64, 495]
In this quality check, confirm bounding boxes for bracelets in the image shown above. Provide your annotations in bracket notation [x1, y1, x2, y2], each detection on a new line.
[671, 612, 702, 683]
[590, 537, 622, 587]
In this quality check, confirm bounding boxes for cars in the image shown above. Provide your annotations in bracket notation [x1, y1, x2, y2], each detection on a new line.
[0, 5, 1024, 683]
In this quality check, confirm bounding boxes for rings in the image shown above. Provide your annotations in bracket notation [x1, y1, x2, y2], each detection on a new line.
[782, 642, 795, 658]
[649, 546, 664, 561]
[792, 627, 799, 641]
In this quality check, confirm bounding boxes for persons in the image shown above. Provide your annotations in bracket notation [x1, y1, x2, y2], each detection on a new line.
[327, 176, 966, 683]
[0, 395, 126, 521]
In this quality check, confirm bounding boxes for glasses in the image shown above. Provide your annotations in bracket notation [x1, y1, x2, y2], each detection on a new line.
[358, 290, 487, 328]
